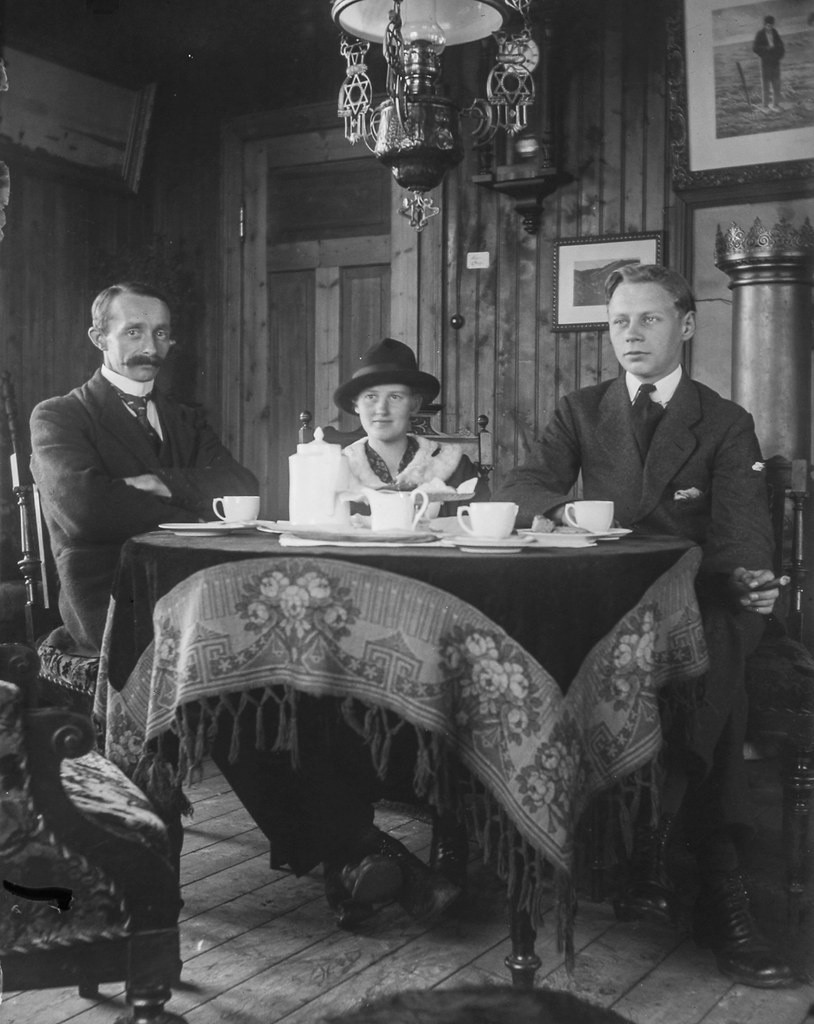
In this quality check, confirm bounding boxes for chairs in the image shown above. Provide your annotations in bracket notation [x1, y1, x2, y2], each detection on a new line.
[0, 637, 187, 1024]
[9, 449, 101, 715]
[597, 452, 814, 904]
[286, 399, 495, 520]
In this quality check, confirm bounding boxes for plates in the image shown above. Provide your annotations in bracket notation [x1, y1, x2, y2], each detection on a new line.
[516, 527, 609, 548]
[590, 528, 632, 541]
[441, 535, 538, 554]
[158, 522, 244, 537]
[257, 527, 284, 534]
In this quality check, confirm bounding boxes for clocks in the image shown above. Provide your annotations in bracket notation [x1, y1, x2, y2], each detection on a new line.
[470, 0, 570, 235]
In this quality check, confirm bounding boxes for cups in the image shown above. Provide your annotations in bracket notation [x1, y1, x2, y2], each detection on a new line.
[565, 499, 614, 535]
[212, 496, 260, 523]
[456, 501, 519, 540]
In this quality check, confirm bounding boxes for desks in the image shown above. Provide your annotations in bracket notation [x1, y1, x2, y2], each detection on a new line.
[94, 518, 710, 988]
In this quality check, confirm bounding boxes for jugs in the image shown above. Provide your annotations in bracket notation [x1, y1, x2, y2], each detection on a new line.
[287, 427, 357, 524]
[361, 484, 429, 531]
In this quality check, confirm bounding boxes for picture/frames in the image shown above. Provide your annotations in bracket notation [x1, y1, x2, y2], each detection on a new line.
[550, 230, 666, 333]
[659, 0, 814, 201]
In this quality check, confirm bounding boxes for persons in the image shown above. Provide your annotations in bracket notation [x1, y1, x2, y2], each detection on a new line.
[752, 17, 786, 115]
[333, 338, 491, 518]
[496, 267, 798, 987]
[29, 282, 457, 926]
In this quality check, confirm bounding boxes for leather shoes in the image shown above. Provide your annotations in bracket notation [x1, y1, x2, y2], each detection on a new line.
[615, 834, 676, 923]
[694, 875, 795, 986]
[384, 837, 462, 926]
[437, 843, 467, 875]
[327, 854, 403, 928]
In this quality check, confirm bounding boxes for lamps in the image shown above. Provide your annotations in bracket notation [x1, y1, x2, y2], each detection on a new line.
[327, 0, 539, 234]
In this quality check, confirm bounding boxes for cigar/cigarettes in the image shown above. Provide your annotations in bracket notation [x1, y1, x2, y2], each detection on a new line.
[752, 575, 790, 592]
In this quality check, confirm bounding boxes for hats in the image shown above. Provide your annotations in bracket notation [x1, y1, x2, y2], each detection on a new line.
[333, 339, 441, 417]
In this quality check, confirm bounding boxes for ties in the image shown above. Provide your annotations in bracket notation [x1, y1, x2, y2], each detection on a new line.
[106, 380, 161, 457]
[630, 384, 657, 462]
